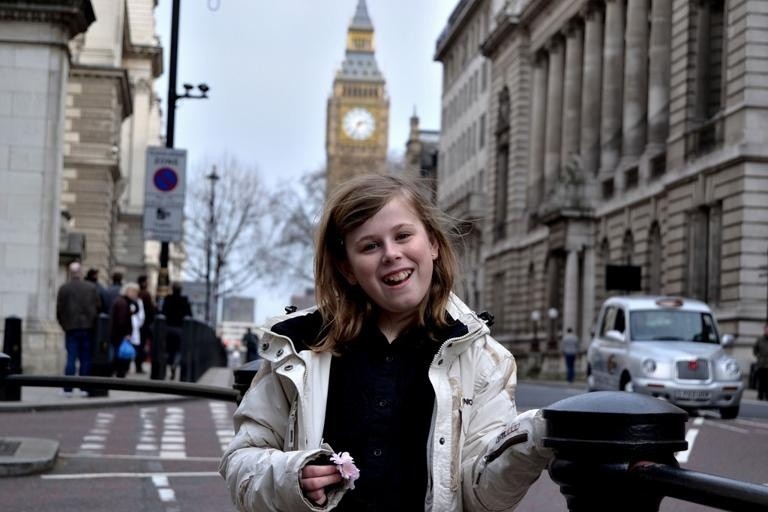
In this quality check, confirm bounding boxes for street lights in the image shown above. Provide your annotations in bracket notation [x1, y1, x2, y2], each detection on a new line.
[547, 308, 559, 350]
[530, 310, 541, 352]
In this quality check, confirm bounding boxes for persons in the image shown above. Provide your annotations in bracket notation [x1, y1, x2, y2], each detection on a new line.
[558, 327, 581, 382]
[218, 172, 553, 512]
[242, 327, 259, 362]
[584, 332, 595, 379]
[751, 325, 768, 401]
[54, 261, 192, 395]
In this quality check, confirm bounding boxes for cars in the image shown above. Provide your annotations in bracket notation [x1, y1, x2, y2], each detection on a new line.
[584, 295, 745, 419]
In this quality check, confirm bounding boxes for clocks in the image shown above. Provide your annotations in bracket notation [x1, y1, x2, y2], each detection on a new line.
[341, 106, 378, 144]
